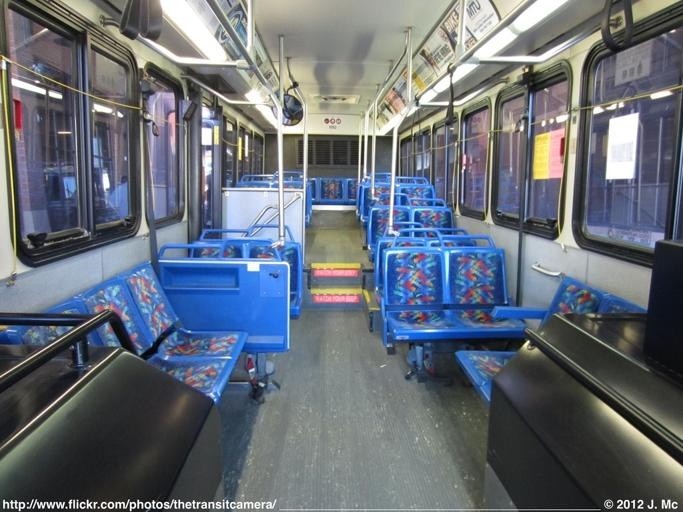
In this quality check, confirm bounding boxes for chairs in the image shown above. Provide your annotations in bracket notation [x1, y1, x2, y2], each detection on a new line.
[236, 170, 355, 229]
[354, 170, 529, 384]
[452, 273, 650, 410]
